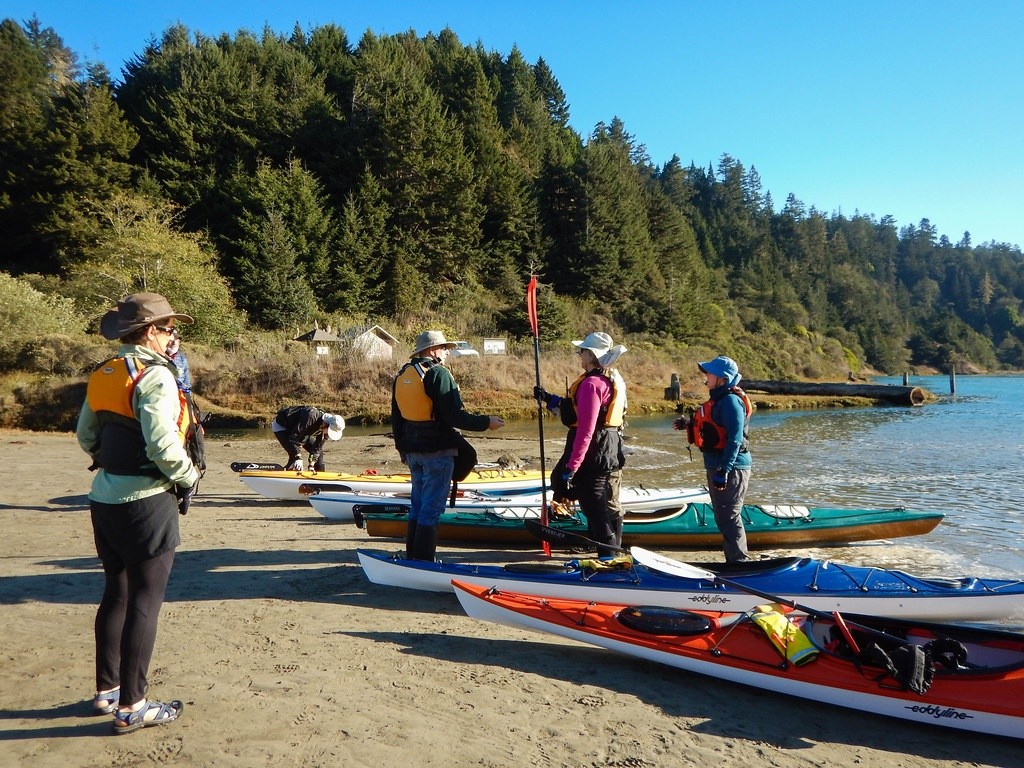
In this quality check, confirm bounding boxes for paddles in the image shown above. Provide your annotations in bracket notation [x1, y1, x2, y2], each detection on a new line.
[527, 276, 552, 557]
[229, 461, 299, 473]
[524, 517, 632, 554]
[296, 482, 356, 494]
[629, 541, 913, 649]
[356, 502, 413, 515]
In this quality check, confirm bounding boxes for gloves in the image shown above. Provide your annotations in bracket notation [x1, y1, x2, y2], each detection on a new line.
[294, 458, 304, 471]
[561, 467, 576, 490]
[307, 465, 317, 475]
[534, 386, 553, 402]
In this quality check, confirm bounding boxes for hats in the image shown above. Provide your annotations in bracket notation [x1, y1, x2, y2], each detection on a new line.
[698, 357, 742, 390]
[101, 292, 194, 340]
[409, 331, 457, 358]
[322, 413, 345, 441]
[571, 331, 628, 370]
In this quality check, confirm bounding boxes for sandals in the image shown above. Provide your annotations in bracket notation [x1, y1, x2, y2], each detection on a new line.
[114, 699, 183, 734]
[93, 690, 122, 714]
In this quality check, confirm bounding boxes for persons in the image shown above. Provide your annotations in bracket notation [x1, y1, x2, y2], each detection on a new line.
[533, 331, 627, 559]
[77, 291, 199, 732]
[673, 355, 753, 563]
[166, 328, 191, 452]
[391, 331, 505, 561]
[272, 405, 345, 474]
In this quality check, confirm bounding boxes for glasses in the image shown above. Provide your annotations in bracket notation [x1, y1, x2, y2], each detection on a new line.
[436, 344, 448, 351]
[580, 348, 590, 353]
[144, 325, 174, 337]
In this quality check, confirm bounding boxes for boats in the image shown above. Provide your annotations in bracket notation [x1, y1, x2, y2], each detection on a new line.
[449, 583, 1024, 746]
[358, 546, 1022, 598]
[308, 490, 715, 522]
[231, 463, 552, 504]
[359, 504, 945, 547]
